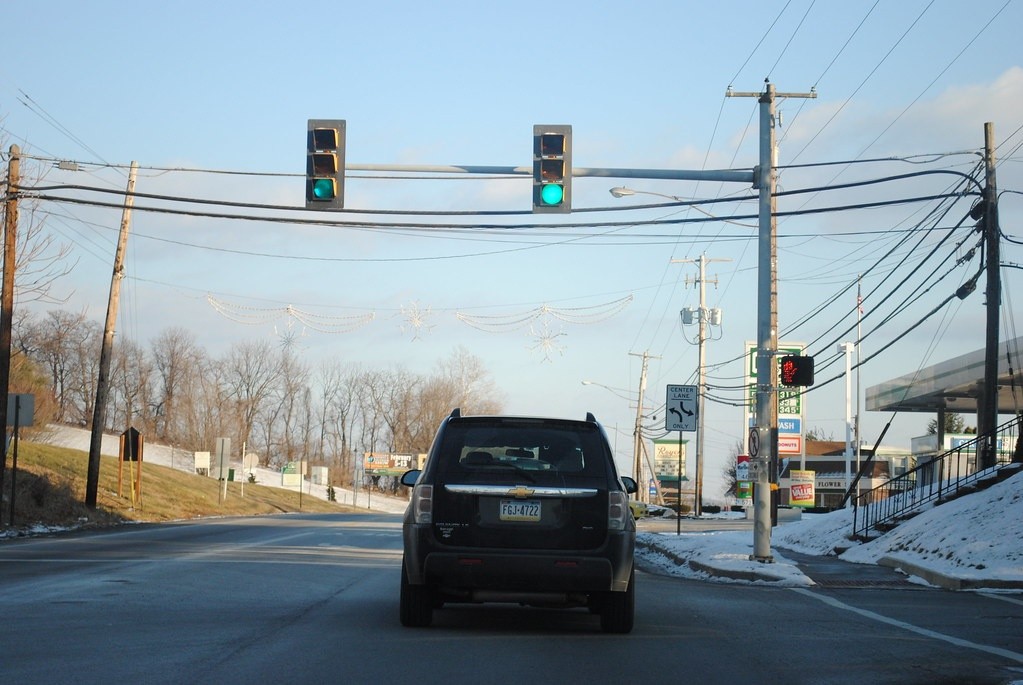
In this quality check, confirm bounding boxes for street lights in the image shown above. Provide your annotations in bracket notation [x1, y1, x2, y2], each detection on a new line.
[609, 186, 779, 525]
[581, 379, 643, 499]
[838, 342, 855, 509]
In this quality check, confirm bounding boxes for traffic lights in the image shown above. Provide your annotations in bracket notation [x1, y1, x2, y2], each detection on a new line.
[532, 125, 572, 213]
[781, 356, 814, 387]
[306, 118, 345, 209]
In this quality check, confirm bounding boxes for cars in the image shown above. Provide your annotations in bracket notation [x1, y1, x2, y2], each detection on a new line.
[629, 500, 649, 520]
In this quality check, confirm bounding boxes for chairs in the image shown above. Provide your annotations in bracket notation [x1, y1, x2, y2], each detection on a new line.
[555, 451, 582, 470]
[461, 451, 495, 466]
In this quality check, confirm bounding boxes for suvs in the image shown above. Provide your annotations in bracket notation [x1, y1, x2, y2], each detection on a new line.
[399, 409, 639, 634]
